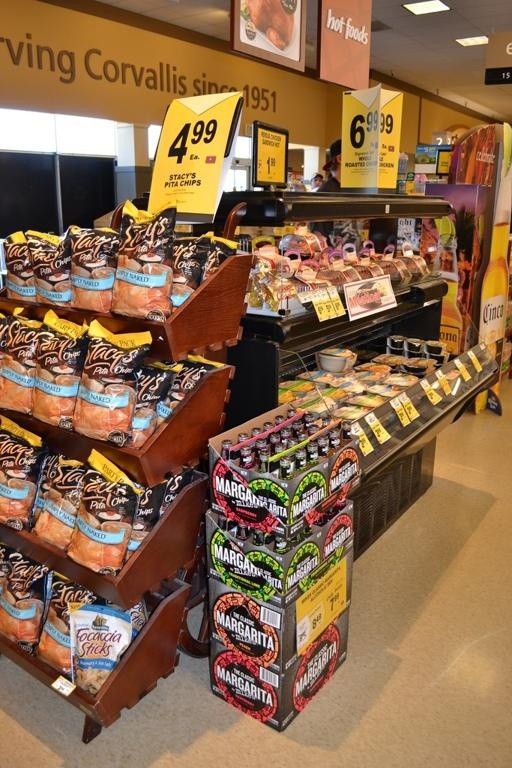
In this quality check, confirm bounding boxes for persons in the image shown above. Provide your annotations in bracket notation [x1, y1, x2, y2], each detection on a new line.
[312, 138, 342, 234]
[310, 174, 323, 192]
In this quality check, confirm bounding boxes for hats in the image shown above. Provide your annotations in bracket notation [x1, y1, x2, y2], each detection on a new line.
[322, 139, 341, 172]
[310, 173, 323, 181]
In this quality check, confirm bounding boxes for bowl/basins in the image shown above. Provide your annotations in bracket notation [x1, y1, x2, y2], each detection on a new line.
[318, 348, 353, 373]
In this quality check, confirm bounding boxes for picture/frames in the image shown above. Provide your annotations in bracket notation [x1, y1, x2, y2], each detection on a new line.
[231, 0, 307, 73]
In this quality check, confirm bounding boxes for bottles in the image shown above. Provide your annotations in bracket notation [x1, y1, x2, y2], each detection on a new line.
[476, 211, 510, 414]
[433, 233, 463, 356]
[218, 409, 352, 554]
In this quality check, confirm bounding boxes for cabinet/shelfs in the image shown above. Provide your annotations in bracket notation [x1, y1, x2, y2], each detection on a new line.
[212, 191, 501, 560]
[0, 207, 252, 744]
